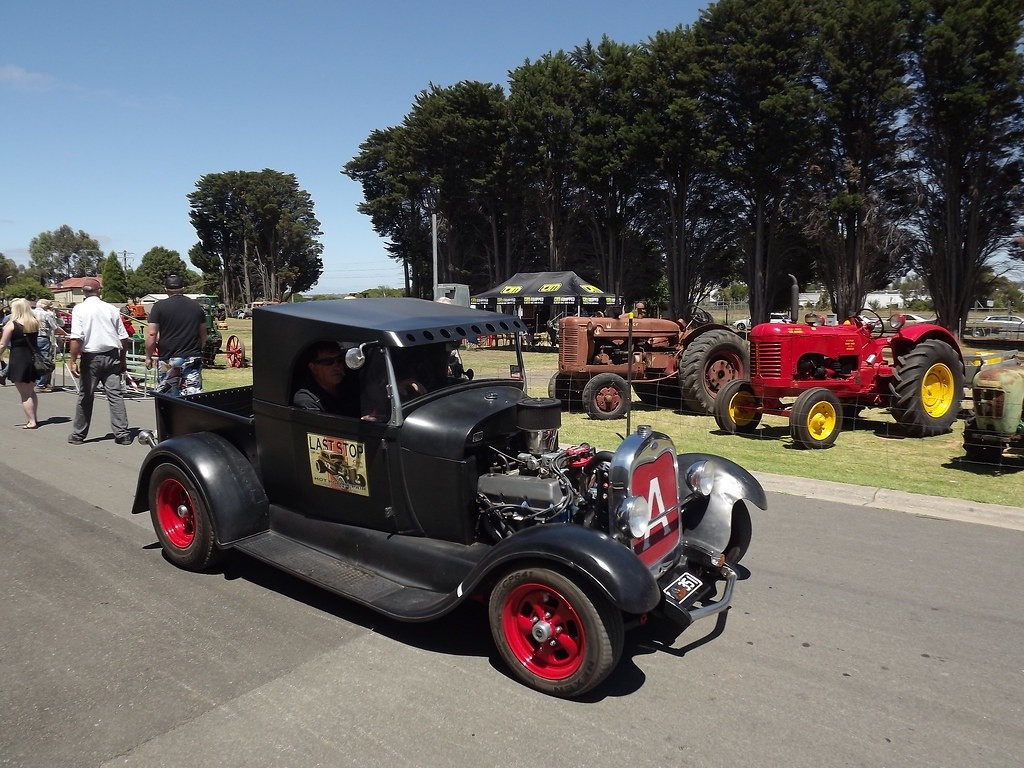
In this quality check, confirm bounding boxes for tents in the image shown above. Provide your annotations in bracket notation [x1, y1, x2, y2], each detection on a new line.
[470, 272, 624, 352]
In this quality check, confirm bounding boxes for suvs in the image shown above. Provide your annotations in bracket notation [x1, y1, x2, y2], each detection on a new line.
[733, 310, 792, 331]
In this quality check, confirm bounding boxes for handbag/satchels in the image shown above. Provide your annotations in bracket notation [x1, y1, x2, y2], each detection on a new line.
[34, 353, 55, 376]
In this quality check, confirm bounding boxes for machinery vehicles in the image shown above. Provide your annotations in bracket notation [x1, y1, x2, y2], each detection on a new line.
[548, 282, 750, 421]
[714, 274, 966, 450]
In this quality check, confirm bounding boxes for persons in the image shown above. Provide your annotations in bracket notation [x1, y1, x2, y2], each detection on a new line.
[293, 340, 426, 422]
[67, 278, 133, 446]
[0, 298, 65, 429]
[144, 274, 207, 397]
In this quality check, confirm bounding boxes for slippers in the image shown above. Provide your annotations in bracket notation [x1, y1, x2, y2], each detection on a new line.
[23, 425, 38, 429]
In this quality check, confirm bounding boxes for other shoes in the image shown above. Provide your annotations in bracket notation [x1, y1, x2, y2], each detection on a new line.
[115, 435, 132, 445]
[67, 435, 84, 444]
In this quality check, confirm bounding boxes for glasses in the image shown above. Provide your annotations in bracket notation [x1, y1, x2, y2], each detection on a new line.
[314, 353, 344, 366]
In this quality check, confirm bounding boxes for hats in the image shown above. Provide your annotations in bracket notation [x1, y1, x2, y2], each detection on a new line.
[165, 275, 182, 289]
[83, 279, 103, 290]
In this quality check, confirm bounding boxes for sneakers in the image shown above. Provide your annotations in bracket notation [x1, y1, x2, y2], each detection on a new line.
[34, 384, 53, 392]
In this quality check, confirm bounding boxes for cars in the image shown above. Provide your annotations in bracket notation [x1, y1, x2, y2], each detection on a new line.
[813, 314, 938, 333]
[964, 315, 1024, 338]
